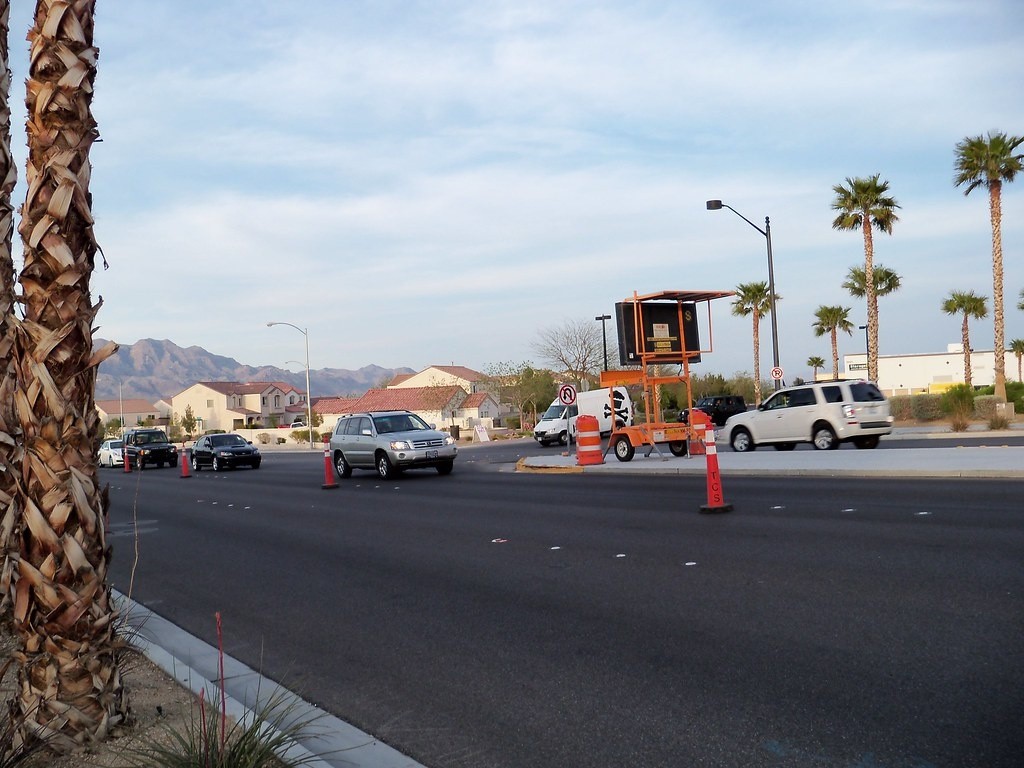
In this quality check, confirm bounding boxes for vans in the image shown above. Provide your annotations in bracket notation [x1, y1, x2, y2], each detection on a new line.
[534, 386, 635, 447]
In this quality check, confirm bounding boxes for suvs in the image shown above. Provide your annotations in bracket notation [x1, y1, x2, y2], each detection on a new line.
[329, 409, 458, 481]
[724, 379, 894, 451]
[121, 428, 178, 470]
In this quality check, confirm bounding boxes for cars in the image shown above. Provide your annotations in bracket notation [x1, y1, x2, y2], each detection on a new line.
[190, 434, 261, 472]
[677, 395, 747, 426]
[96, 440, 125, 468]
[275, 424, 290, 428]
[290, 422, 306, 428]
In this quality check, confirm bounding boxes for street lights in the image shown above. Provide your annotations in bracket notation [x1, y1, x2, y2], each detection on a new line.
[859, 325, 868, 357]
[119, 381, 123, 439]
[707, 200, 783, 405]
[267, 322, 313, 451]
[595, 315, 612, 372]
